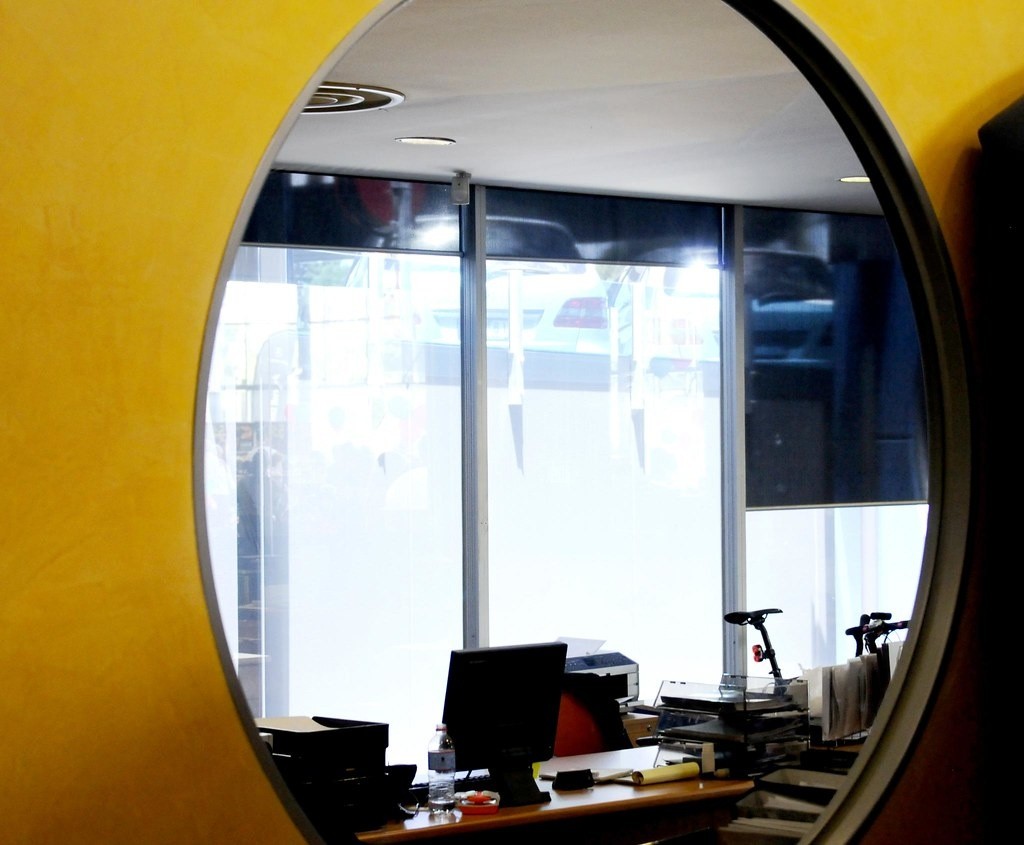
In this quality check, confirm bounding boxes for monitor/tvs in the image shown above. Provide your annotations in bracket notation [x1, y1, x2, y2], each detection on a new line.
[433, 641, 569, 809]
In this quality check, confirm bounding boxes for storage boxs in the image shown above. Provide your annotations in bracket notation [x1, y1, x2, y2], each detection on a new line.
[258, 716, 389, 833]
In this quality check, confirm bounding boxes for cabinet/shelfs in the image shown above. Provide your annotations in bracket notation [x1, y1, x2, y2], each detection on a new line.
[620, 712, 659, 749]
[650, 673, 812, 778]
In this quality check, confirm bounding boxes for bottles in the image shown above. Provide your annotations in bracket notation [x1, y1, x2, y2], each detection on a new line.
[426, 721, 457, 816]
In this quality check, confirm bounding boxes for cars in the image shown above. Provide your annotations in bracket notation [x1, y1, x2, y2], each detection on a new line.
[743, 249, 840, 384]
[365, 211, 585, 260]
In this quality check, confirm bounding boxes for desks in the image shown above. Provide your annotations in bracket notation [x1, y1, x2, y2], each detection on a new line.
[355, 745, 754, 845]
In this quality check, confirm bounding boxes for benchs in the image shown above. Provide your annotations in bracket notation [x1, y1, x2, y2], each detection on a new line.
[553, 673, 634, 756]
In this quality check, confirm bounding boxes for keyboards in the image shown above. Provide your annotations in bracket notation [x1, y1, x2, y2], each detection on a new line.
[403, 772, 495, 805]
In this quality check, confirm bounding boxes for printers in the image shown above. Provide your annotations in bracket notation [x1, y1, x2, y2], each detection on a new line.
[564, 651, 644, 714]
